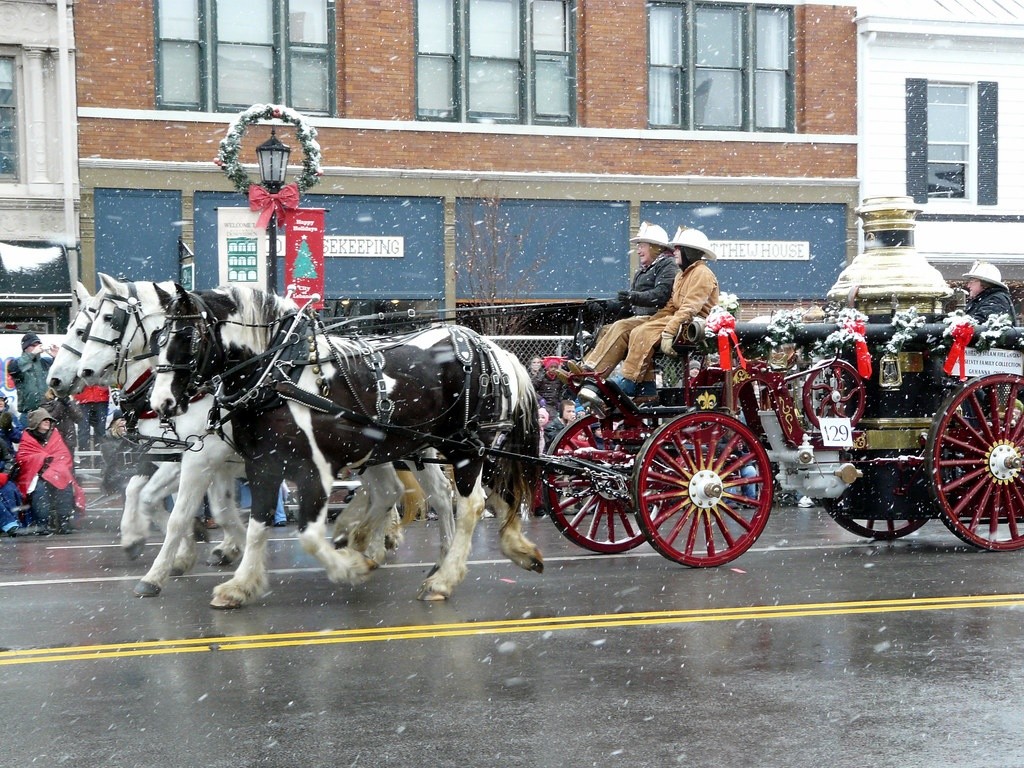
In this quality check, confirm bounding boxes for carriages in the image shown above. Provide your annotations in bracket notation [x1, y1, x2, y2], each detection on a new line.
[45, 273, 1024, 608]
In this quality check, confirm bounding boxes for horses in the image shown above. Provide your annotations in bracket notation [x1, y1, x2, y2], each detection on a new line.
[46, 273, 545, 612]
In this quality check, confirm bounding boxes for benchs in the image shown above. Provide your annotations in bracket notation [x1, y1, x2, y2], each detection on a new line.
[658, 296, 737, 352]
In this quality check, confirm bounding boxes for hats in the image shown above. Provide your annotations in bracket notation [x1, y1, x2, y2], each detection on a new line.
[0, 390, 6, 397]
[21, 333, 42, 351]
[543, 356, 562, 368]
[668, 227, 717, 262]
[689, 359, 701, 370]
[962, 261, 1010, 291]
[25, 409, 57, 430]
[630, 220, 672, 251]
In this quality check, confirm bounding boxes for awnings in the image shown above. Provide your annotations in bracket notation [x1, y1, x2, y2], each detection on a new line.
[0, 239, 73, 307]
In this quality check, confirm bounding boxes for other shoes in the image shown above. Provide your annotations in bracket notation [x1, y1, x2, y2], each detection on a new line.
[555, 368, 570, 385]
[533, 508, 545, 516]
[480, 509, 494, 519]
[727, 501, 740, 508]
[38, 519, 53, 535]
[604, 373, 659, 402]
[10, 523, 39, 535]
[798, 495, 815, 508]
[567, 359, 584, 376]
[563, 505, 580, 514]
[59, 518, 72, 534]
[204, 517, 218, 529]
[276, 521, 287, 527]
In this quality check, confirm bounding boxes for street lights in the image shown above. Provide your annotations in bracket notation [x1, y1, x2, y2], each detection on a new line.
[254, 124, 292, 294]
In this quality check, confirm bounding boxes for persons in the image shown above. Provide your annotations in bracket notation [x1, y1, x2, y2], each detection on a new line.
[526, 355, 615, 456]
[933, 261, 1019, 327]
[555, 221, 680, 385]
[173, 476, 290, 527]
[776, 463, 816, 508]
[0, 391, 87, 535]
[715, 427, 756, 508]
[606, 225, 719, 407]
[8, 332, 140, 505]
